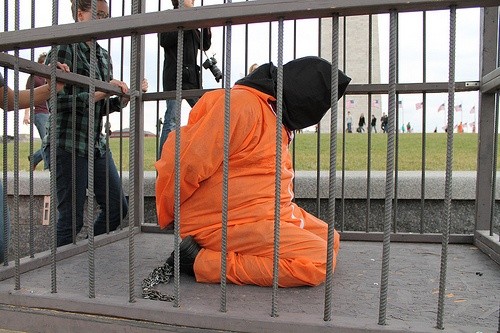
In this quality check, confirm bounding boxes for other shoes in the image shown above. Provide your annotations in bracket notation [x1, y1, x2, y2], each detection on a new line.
[28, 156, 36, 170]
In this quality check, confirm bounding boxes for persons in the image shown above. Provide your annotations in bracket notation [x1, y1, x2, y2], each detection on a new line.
[407, 122, 411, 133]
[157, 57, 351, 288]
[402, 124, 405, 133]
[104, 120, 112, 139]
[0, 62, 69, 261]
[358, 113, 366, 133]
[458, 122, 463, 132]
[249, 64, 258, 74]
[347, 111, 353, 133]
[44, 0, 147, 246]
[23, 54, 50, 171]
[371, 115, 377, 133]
[381, 112, 388, 133]
[157, 0, 211, 153]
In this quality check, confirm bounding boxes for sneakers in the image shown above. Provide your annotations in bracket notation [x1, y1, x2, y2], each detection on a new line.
[165, 236, 201, 266]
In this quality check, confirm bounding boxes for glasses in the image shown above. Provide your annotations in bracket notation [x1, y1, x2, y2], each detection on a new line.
[84, 10, 109, 20]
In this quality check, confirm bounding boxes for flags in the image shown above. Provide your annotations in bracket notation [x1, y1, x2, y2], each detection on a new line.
[416, 102, 423, 109]
[469, 106, 475, 113]
[438, 104, 445, 112]
[347, 100, 402, 109]
[454, 104, 462, 112]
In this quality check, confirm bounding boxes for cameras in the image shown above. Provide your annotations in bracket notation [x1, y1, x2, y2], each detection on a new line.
[203, 57, 223, 83]
